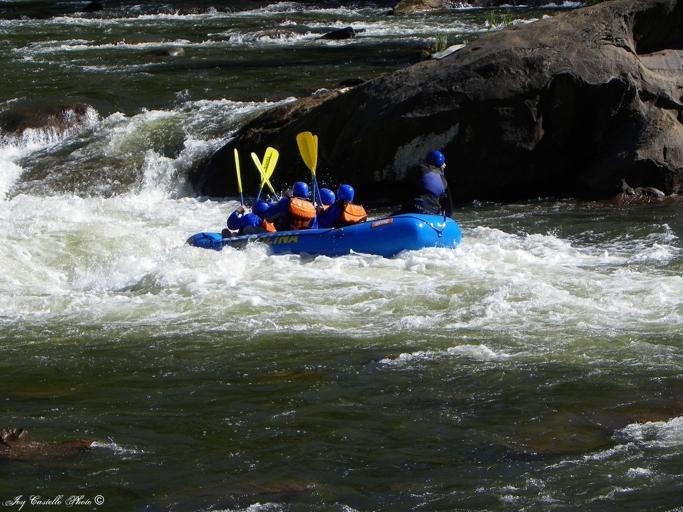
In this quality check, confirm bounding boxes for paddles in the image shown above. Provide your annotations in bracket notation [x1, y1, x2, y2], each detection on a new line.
[250, 151, 279, 202]
[234, 147, 244, 216]
[256, 146, 279, 203]
[311, 135, 318, 208]
[296, 130, 324, 210]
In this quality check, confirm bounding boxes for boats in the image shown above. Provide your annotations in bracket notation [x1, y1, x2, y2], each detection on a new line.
[183, 212, 461, 258]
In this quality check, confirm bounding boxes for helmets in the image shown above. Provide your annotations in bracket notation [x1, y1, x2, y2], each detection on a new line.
[337, 184, 355, 202]
[292, 181, 309, 198]
[427, 151, 445, 167]
[252, 195, 289, 218]
[317, 187, 336, 204]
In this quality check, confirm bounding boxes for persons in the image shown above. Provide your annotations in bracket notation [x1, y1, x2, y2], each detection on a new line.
[387, 149, 453, 217]
[222, 182, 368, 239]
